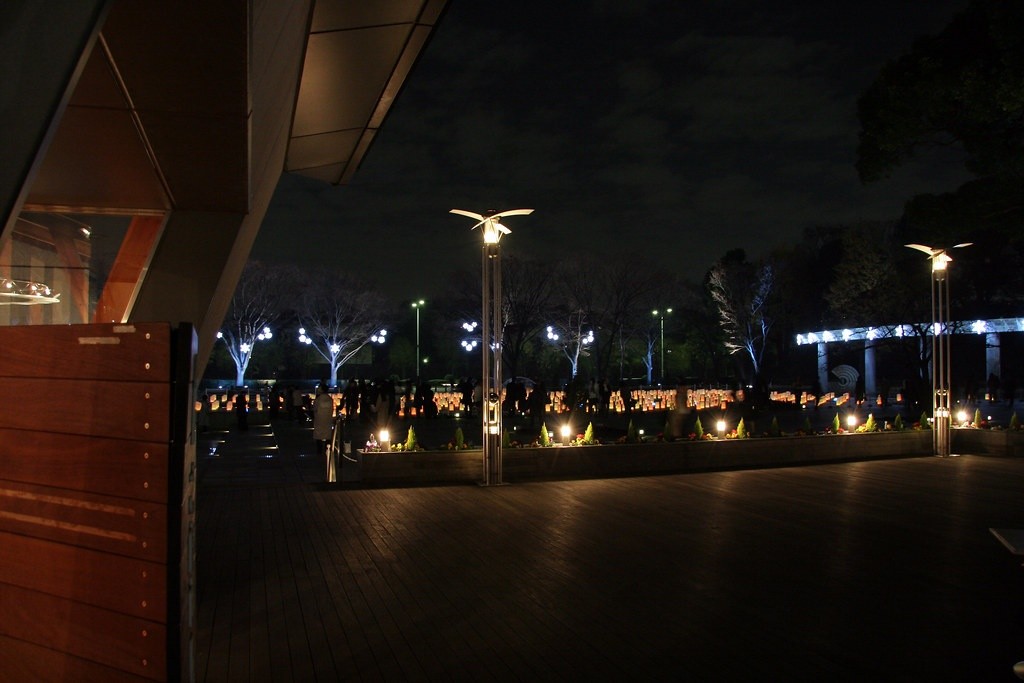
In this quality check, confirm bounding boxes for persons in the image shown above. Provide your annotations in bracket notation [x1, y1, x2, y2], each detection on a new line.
[196, 372, 1017, 431]
[311, 383, 334, 472]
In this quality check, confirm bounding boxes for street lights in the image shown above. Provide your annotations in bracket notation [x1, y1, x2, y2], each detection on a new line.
[411, 300, 426, 390]
[905, 241, 974, 458]
[653, 308, 673, 380]
[449, 207, 535, 486]
